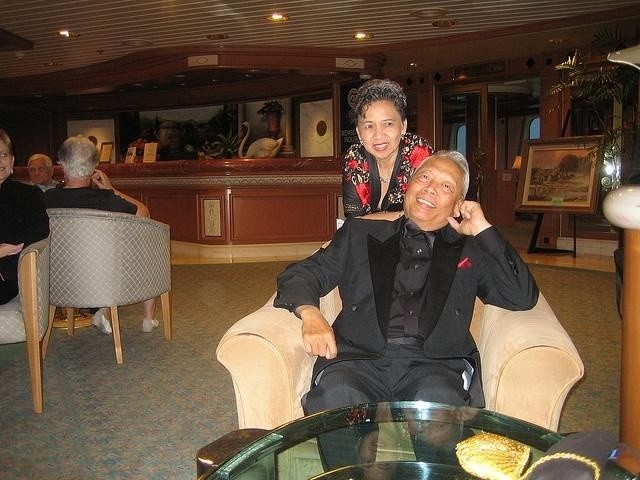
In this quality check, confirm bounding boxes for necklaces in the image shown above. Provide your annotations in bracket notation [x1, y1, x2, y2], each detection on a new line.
[378, 176, 392, 187]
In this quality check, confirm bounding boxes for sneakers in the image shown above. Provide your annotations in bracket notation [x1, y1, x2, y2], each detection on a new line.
[92, 312, 113, 335]
[142, 318, 160, 333]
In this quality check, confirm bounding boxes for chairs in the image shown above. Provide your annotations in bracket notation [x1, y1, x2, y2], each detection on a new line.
[46, 206, 176, 362]
[216, 240, 586, 474]
[0, 232, 54, 415]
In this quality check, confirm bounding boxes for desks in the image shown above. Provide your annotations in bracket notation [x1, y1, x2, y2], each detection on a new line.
[197, 400, 637, 480]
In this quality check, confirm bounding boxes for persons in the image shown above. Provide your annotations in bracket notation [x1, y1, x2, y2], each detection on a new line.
[273, 149, 540, 471]
[0, 129, 160, 335]
[343, 78, 434, 221]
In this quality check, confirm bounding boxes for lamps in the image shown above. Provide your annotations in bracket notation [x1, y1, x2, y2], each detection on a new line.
[512, 155, 522, 185]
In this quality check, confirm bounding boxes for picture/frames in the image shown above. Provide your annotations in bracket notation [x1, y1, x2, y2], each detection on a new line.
[99, 142, 113, 162]
[515, 136, 605, 213]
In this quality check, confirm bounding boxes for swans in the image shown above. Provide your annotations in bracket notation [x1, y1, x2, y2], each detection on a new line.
[238, 122, 284, 160]
